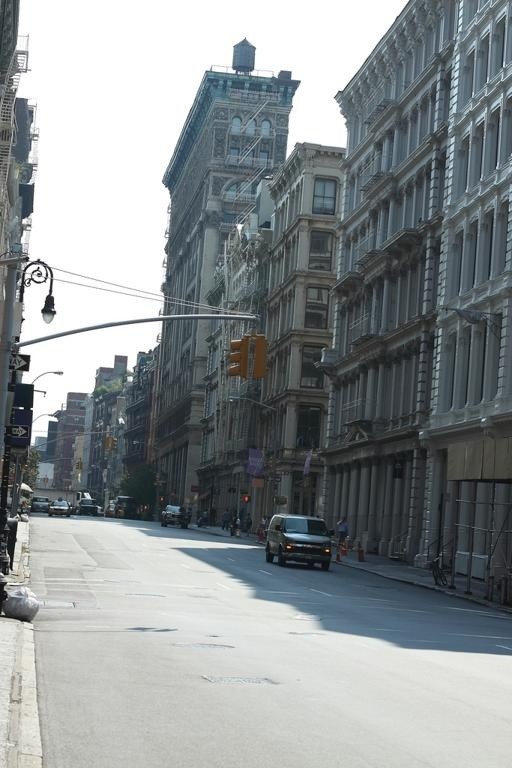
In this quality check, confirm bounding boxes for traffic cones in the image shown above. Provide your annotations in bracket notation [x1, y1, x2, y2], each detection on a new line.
[336, 548, 342, 561]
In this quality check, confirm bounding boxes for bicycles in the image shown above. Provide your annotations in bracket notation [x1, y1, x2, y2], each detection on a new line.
[431, 553, 448, 585]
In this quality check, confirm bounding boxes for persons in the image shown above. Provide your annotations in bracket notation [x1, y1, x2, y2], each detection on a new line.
[256, 514, 273, 544]
[187, 507, 252, 537]
[336, 516, 348, 552]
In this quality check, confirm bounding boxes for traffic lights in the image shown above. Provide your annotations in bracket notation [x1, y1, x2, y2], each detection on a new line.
[76, 461, 83, 470]
[106, 436, 117, 449]
[227, 334, 268, 379]
[244, 495, 251, 503]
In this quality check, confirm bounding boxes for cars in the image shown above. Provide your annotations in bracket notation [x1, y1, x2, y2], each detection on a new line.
[162, 504, 190, 530]
[106, 497, 137, 518]
[31, 495, 98, 517]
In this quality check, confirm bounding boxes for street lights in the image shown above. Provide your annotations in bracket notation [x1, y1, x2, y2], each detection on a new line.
[0, 258, 56, 574]
[228, 396, 277, 412]
[31, 371, 63, 383]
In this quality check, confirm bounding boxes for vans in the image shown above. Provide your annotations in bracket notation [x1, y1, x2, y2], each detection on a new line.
[265, 513, 332, 571]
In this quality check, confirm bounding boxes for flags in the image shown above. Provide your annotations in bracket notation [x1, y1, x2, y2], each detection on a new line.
[303, 448, 313, 475]
[245, 447, 266, 478]
